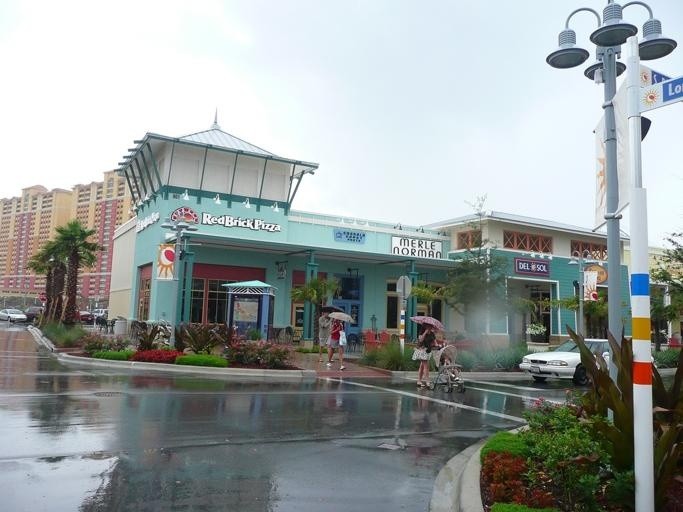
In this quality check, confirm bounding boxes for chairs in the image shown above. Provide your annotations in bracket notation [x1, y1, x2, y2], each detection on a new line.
[417, 382, 430, 387]
[318, 358, 345, 370]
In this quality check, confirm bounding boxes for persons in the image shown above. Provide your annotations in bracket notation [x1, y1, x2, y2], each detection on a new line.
[416, 323, 442, 390]
[327, 319, 346, 370]
[442, 355, 460, 381]
[318, 311, 335, 362]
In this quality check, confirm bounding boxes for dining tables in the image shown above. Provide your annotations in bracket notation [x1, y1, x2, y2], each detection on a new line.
[115, 316, 127, 335]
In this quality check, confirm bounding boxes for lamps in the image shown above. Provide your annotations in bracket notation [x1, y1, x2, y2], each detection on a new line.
[345, 330, 399, 353]
[270, 326, 304, 345]
[96, 316, 118, 333]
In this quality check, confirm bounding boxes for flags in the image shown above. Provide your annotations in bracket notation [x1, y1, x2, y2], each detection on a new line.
[530, 334, 549, 343]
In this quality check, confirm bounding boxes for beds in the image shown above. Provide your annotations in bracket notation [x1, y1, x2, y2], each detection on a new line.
[320, 304, 345, 312]
[409, 316, 443, 332]
[328, 311, 354, 324]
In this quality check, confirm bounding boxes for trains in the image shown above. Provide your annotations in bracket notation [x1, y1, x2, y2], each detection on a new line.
[38, 292, 47, 302]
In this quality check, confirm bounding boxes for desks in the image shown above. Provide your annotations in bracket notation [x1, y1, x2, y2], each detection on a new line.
[161, 211, 198, 349]
[545, 0, 678, 424]
[568, 247, 595, 336]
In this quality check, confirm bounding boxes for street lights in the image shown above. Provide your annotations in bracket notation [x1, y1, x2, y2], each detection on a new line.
[339, 331, 347, 346]
[411, 346, 430, 361]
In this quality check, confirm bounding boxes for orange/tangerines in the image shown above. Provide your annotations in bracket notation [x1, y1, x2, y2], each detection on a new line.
[431, 344, 465, 394]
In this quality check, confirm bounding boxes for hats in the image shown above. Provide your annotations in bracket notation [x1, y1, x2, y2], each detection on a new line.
[93, 308, 108, 319]
[26, 305, 45, 322]
[0, 308, 27, 322]
[520, 338, 659, 388]
[80, 310, 94, 322]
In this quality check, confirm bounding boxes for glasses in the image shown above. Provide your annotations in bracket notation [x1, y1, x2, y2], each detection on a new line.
[522, 249, 553, 261]
[438, 229, 447, 237]
[393, 223, 403, 230]
[127, 192, 154, 215]
[213, 194, 222, 205]
[275, 261, 289, 279]
[416, 226, 424, 233]
[271, 202, 279, 212]
[180, 189, 190, 201]
[242, 198, 251, 209]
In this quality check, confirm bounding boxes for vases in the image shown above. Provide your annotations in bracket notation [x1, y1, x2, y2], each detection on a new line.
[525, 323, 547, 335]
